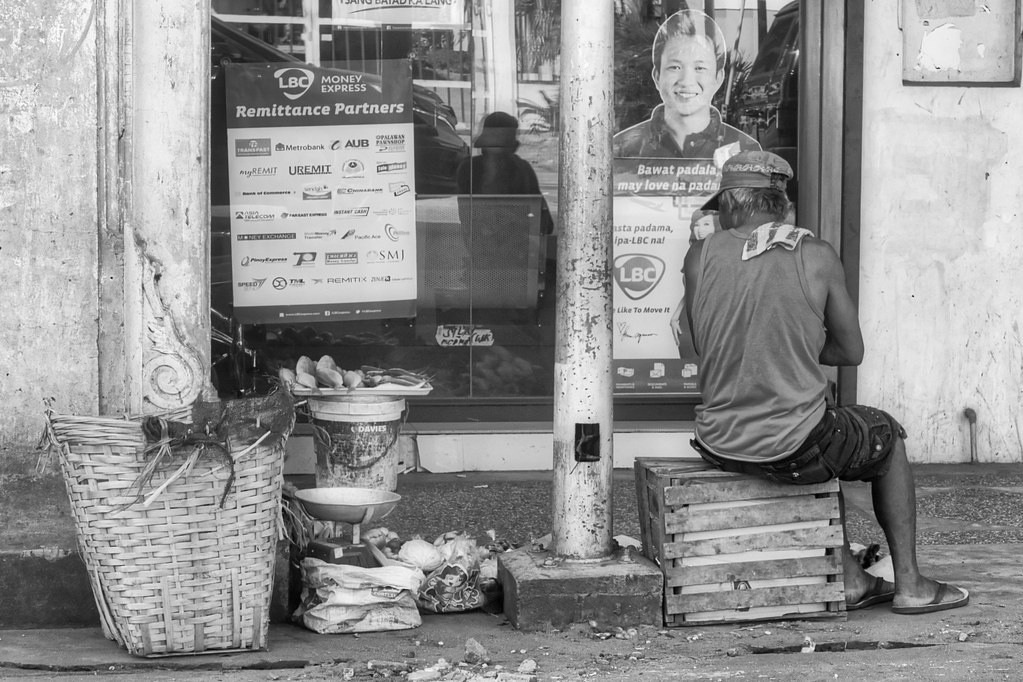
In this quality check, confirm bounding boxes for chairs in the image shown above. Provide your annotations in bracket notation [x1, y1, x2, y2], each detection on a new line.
[415, 192, 551, 344]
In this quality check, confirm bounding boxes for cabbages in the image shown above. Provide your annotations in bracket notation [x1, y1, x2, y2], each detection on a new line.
[396, 527, 505, 579]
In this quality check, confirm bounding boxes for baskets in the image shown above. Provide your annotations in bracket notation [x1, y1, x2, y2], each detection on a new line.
[33, 381, 307, 657]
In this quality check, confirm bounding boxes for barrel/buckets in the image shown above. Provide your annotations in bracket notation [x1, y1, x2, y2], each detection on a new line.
[306, 396, 410, 491]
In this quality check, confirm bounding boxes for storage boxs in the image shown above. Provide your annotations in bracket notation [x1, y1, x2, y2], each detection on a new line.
[633, 456, 848, 625]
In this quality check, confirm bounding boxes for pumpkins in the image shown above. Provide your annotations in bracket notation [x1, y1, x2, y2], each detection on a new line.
[361, 527, 403, 555]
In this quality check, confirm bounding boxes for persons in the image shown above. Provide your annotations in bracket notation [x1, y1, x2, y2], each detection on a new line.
[686, 152, 970, 614]
[458, 110, 555, 319]
[613, 9, 762, 194]
[671, 210, 723, 359]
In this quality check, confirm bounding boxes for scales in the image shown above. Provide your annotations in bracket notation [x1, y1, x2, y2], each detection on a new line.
[294, 487, 402, 633]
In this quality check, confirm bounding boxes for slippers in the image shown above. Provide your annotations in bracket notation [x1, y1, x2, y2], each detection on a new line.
[846, 576, 895, 609]
[892, 582, 970, 613]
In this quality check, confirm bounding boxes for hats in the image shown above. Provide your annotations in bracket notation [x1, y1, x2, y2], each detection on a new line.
[699, 151, 794, 210]
[474, 112, 518, 148]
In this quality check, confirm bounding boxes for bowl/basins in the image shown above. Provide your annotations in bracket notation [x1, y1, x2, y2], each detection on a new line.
[294, 487, 403, 525]
